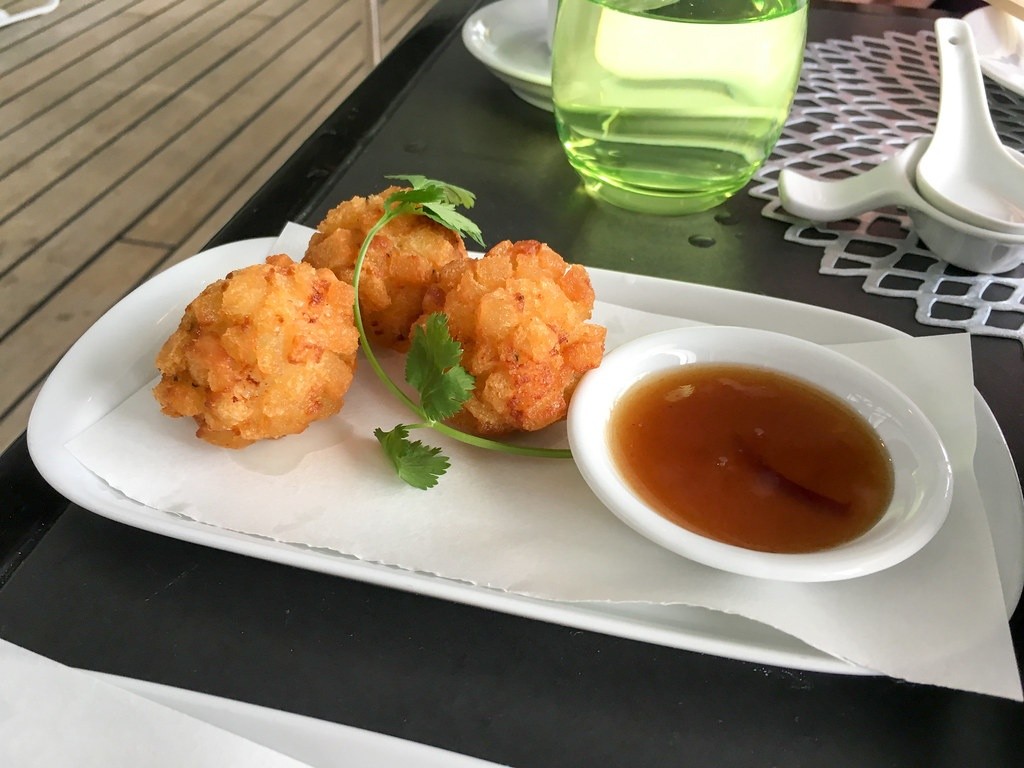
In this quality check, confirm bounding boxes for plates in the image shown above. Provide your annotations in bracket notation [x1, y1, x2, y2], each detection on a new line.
[461, 1, 559, 113]
[26, 234, 1024, 677]
[962, 5, 1024, 98]
[566, 325, 954, 584]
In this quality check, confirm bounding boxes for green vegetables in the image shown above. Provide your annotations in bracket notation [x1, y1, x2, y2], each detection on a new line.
[350, 174, 577, 492]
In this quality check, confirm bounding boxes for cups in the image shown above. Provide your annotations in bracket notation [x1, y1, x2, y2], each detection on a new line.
[552, 0, 808, 217]
[777, 134, 1024, 274]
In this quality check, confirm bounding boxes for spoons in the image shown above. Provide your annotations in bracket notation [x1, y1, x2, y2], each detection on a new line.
[915, 16, 1024, 234]
